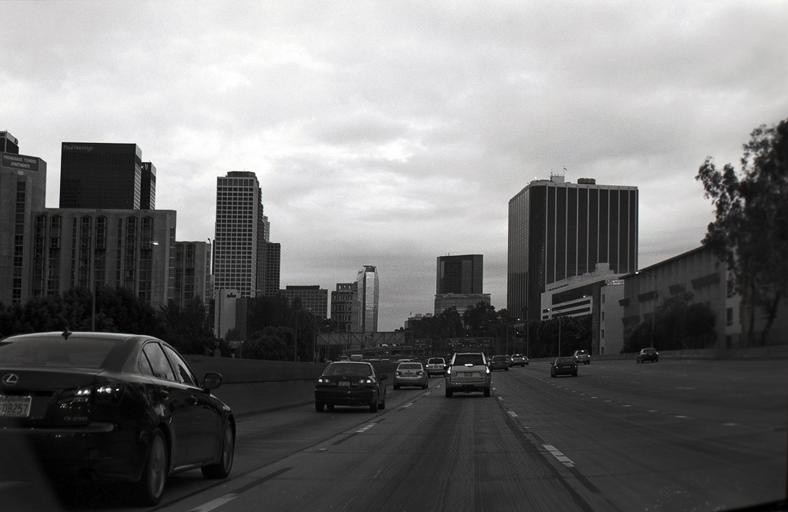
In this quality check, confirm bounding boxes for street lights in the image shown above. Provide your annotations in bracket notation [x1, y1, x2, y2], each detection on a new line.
[547, 308, 561, 358]
[294, 307, 313, 362]
[635, 269, 655, 347]
[518, 318, 529, 358]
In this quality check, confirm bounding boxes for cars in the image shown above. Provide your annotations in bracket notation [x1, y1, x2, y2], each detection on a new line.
[0, 331, 235, 506]
[550, 349, 591, 377]
[637, 347, 660, 363]
[315, 360, 386, 412]
[392, 352, 530, 398]
